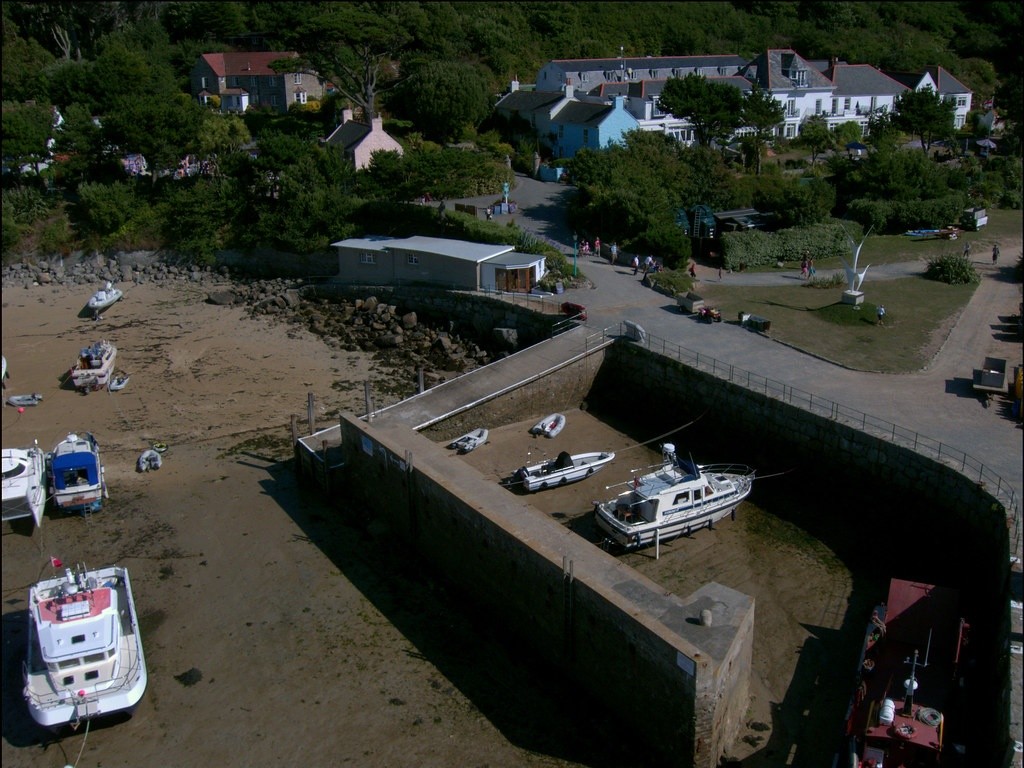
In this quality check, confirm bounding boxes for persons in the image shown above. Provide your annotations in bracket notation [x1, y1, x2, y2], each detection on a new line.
[632, 255, 640, 275]
[799, 254, 815, 280]
[964, 242, 970, 257]
[611, 242, 617, 265]
[992, 245, 1000, 265]
[422, 197, 425, 204]
[719, 267, 722, 281]
[486, 206, 492, 220]
[94, 308, 98, 321]
[691, 263, 696, 277]
[440, 198, 444, 205]
[643, 255, 662, 272]
[579, 240, 590, 256]
[594, 237, 600, 257]
[876, 304, 885, 325]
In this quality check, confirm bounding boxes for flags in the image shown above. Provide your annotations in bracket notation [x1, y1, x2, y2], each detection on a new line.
[984, 100, 992, 106]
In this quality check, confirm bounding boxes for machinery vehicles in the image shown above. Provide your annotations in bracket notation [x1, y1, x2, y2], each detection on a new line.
[697, 305, 722, 324]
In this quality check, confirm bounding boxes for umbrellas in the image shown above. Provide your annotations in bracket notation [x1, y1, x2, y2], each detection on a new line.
[845, 142, 867, 159]
[976, 138, 996, 152]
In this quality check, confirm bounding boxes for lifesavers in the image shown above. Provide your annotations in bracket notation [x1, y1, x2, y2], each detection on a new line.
[154, 443, 168, 453]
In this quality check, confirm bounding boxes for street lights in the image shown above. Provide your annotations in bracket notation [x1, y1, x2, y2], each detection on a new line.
[571, 230, 578, 278]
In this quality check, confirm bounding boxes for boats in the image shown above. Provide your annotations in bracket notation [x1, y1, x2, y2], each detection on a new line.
[49, 430, 110, 518]
[86, 280, 124, 321]
[590, 442, 757, 553]
[139, 450, 162, 472]
[7, 393, 43, 407]
[531, 412, 567, 440]
[109, 375, 130, 392]
[2, 437, 48, 531]
[21, 555, 148, 737]
[827, 573, 975, 767]
[446, 426, 488, 453]
[69, 338, 118, 396]
[511, 450, 617, 493]
